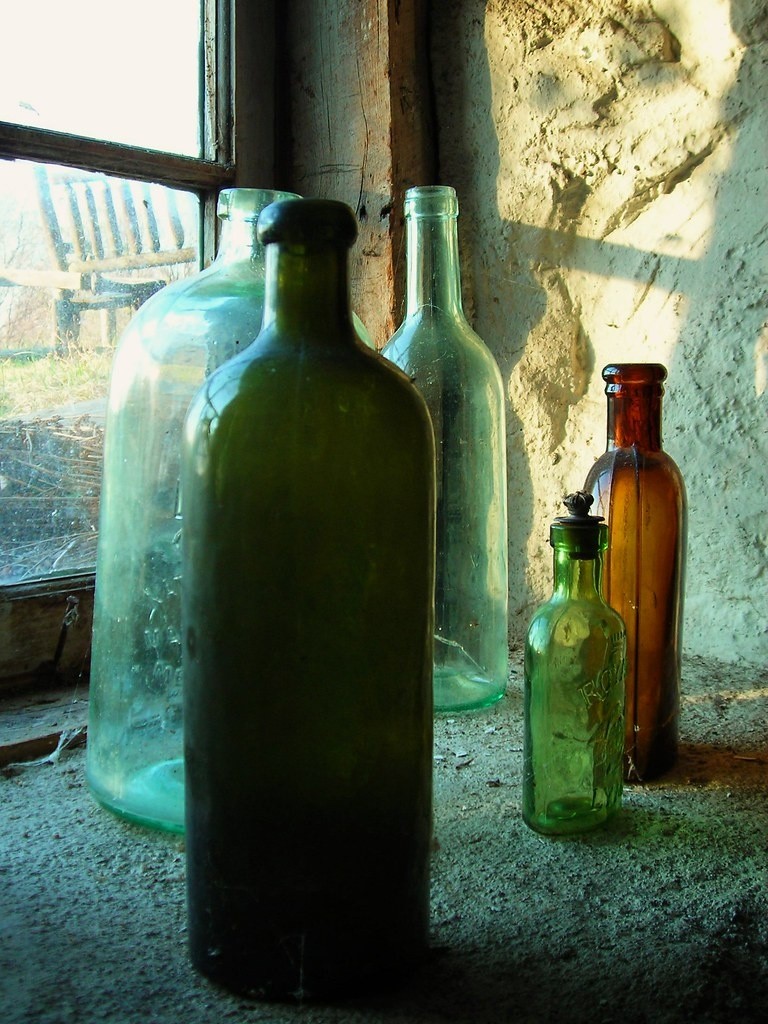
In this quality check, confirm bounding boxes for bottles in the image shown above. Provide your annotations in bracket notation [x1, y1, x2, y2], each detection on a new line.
[525, 490, 626, 835]
[88, 187, 303, 833]
[379, 187, 508, 717]
[583, 364, 688, 785]
[186, 200, 437, 1003]
